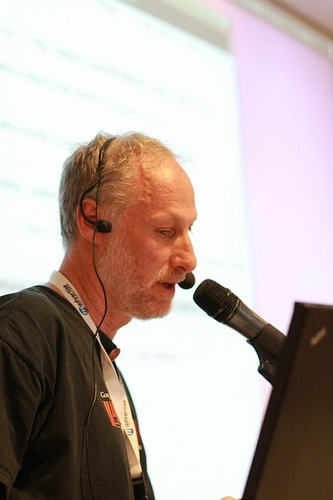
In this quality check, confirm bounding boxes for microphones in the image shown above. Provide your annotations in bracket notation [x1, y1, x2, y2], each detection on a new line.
[178, 272, 196, 290]
[193, 279, 287, 362]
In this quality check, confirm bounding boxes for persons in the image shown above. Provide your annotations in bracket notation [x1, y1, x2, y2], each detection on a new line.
[0, 125, 238, 500]
